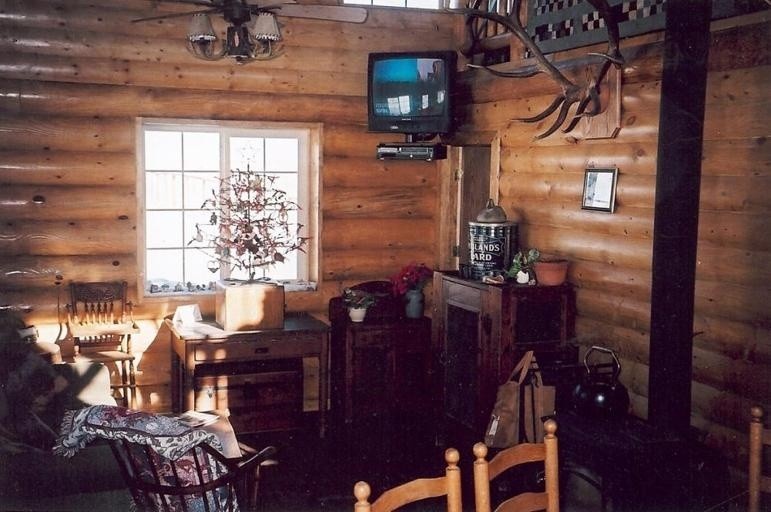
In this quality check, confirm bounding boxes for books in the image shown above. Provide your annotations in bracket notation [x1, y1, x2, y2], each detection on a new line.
[174, 410, 220, 430]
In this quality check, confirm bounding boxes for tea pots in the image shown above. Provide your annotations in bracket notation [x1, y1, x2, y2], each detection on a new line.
[571, 344, 630, 421]
[476, 197, 507, 224]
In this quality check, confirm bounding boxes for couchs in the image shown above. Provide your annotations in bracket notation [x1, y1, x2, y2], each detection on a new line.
[0, 362, 136, 512]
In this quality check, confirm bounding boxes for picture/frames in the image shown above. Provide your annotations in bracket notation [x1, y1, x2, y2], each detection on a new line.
[581, 169, 619, 213]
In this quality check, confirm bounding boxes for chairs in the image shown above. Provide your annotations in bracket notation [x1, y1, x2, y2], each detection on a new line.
[103, 406, 276, 512]
[65, 281, 141, 412]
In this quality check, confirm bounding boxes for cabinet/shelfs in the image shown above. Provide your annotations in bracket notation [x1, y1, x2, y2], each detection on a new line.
[432, 268, 577, 458]
[332, 315, 432, 429]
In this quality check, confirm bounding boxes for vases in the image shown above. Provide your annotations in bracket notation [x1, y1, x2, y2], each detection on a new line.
[405, 289, 426, 320]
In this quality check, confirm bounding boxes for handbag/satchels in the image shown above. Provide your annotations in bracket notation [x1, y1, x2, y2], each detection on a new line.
[524, 355, 556, 443]
[485, 351, 533, 448]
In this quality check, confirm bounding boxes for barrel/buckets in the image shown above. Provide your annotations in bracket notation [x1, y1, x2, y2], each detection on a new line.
[468, 221, 518, 281]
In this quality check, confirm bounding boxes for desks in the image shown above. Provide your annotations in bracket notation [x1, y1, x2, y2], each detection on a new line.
[165, 311, 330, 440]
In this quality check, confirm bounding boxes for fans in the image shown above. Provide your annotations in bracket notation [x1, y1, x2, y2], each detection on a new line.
[130, 0, 368, 26]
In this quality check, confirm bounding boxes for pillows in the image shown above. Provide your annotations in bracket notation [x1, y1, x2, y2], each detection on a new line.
[1, 350, 73, 451]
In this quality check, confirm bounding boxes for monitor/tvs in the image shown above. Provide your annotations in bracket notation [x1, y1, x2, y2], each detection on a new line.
[367, 49, 458, 142]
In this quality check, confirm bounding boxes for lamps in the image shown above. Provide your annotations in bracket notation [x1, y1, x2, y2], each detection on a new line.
[185, 0, 286, 65]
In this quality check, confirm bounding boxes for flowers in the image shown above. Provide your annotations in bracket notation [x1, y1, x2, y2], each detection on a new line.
[386, 258, 433, 297]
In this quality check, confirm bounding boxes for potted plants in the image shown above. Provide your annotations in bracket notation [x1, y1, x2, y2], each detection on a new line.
[342, 286, 373, 322]
[508, 246, 569, 285]
[186, 161, 316, 333]
